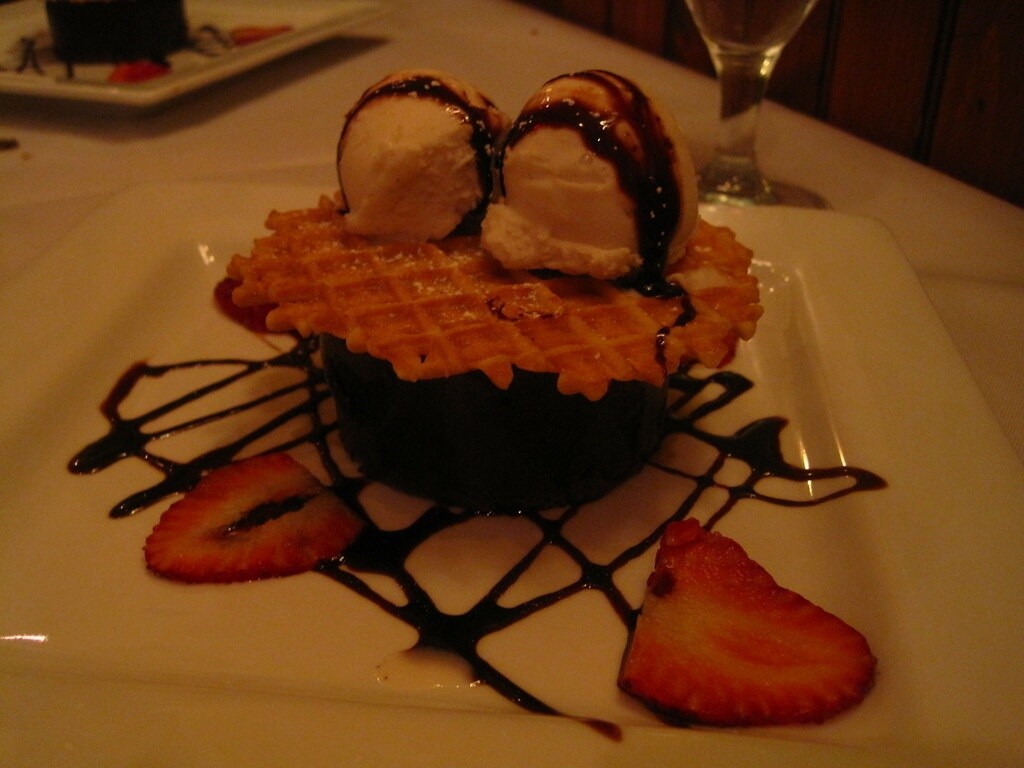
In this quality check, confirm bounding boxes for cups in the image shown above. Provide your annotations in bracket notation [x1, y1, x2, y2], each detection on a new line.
[685, 0, 819, 209]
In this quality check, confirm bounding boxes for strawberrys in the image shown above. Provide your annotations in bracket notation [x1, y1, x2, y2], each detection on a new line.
[109, 60, 170, 84]
[143, 451, 362, 582]
[229, 24, 292, 44]
[618, 517, 879, 725]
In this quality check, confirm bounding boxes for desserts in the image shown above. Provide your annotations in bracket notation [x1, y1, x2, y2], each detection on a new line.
[225, 71, 770, 513]
[45, 0, 191, 62]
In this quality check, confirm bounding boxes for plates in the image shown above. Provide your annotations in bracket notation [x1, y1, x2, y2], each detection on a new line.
[0, 0, 390, 107]
[0, 180, 1024, 768]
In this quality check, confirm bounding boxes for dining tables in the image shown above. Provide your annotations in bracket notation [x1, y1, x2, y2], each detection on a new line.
[0, 0, 1024, 767]
